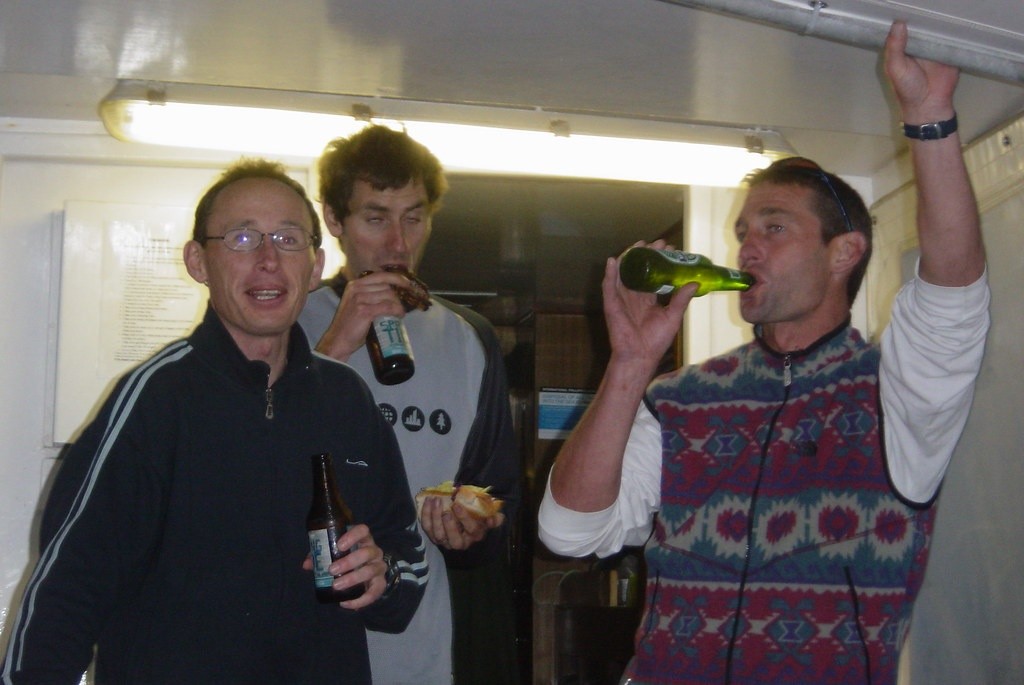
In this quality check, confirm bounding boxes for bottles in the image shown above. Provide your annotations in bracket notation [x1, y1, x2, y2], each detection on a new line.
[305, 453, 364, 605]
[619, 245, 756, 296]
[357, 270, 416, 385]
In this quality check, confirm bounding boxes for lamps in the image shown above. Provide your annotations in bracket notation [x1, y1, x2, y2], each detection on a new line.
[100, 78, 798, 188]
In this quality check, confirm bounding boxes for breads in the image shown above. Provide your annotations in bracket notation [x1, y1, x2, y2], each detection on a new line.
[418, 482, 504, 519]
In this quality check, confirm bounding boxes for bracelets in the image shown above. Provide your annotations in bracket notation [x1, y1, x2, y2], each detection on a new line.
[900, 109, 958, 140]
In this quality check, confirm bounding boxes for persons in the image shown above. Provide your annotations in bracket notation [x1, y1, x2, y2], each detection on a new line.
[297, 125, 521, 685]
[538, 18, 990, 685]
[0, 154, 429, 685]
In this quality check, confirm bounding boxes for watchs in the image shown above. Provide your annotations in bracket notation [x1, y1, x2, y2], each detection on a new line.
[381, 553, 400, 594]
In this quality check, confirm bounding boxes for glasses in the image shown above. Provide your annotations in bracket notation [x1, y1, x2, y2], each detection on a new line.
[200, 229, 319, 252]
[772, 157, 853, 232]
